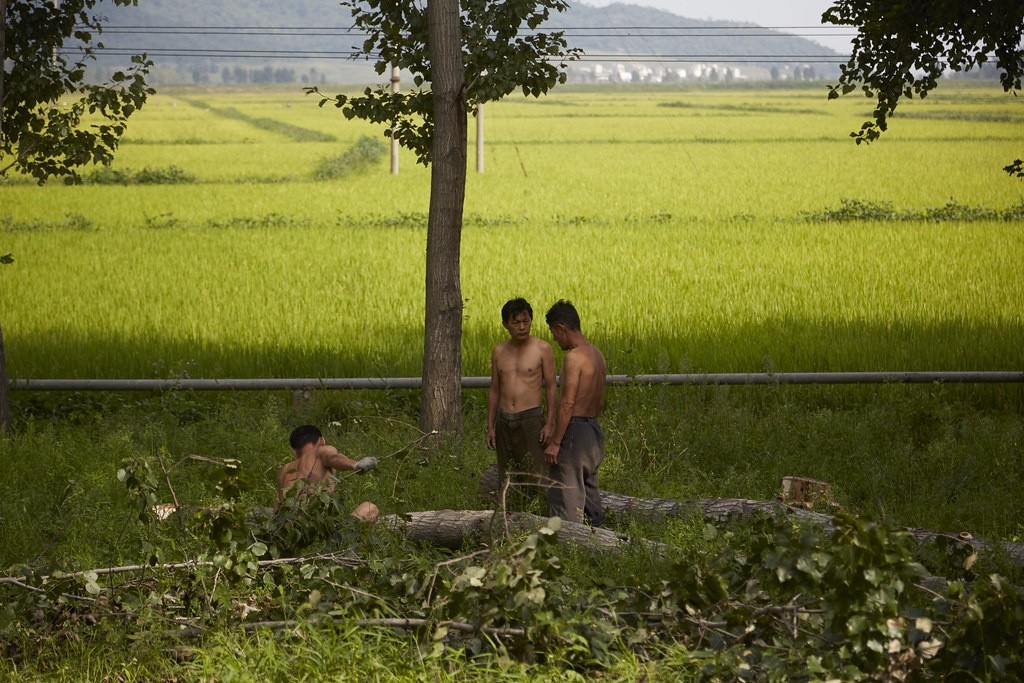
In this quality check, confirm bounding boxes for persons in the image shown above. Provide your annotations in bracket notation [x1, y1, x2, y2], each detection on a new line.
[486, 297, 556, 516]
[274, 425, 379, 523]
[544, 299, 606, 526]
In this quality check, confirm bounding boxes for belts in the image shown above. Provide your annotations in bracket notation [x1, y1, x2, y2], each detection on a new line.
[496, 407, 544, 421]
[567, 416, 595, 424]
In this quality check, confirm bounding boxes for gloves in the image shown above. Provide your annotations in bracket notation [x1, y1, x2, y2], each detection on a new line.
[354, 457, 379, 476]
